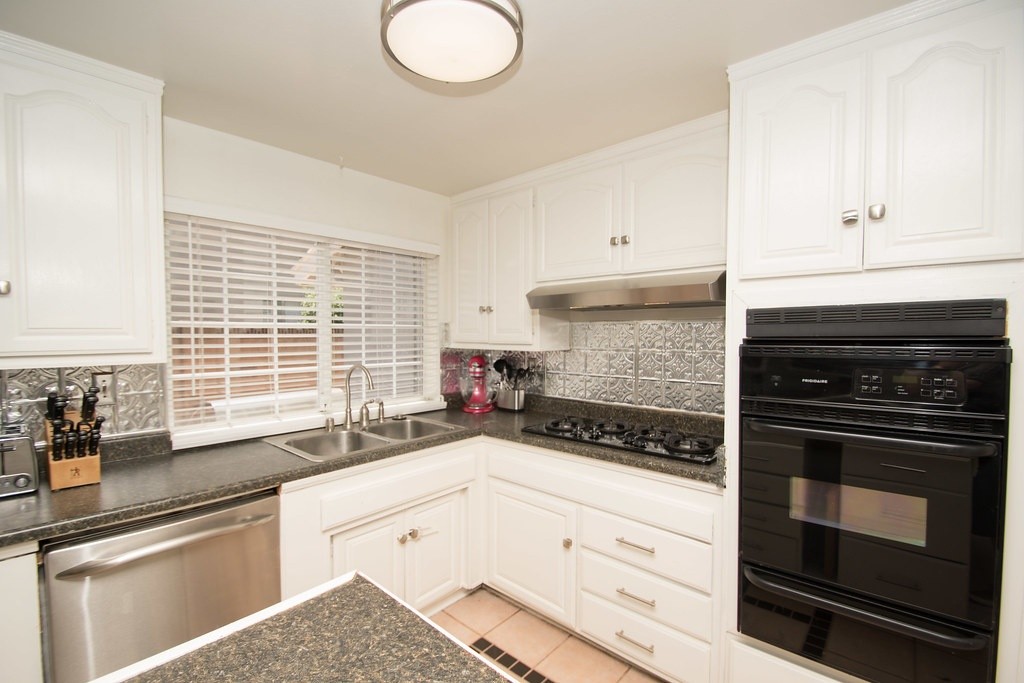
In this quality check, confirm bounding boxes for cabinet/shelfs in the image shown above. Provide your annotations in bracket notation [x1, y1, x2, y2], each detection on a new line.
[0, 30, 166, 368]
[279, 1, 1024, 683]
[0, 540, 48, 683]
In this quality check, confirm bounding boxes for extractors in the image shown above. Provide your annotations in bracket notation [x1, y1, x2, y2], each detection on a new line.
[525, 269, 726, 322]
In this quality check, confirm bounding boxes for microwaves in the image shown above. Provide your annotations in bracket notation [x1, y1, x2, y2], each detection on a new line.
[0, 422, 40, 497]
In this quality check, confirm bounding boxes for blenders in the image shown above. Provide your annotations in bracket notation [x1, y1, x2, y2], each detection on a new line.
[458, 354, 499, 413]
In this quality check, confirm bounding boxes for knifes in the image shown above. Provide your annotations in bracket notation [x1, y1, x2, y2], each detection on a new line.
[44, 386, 106, 462]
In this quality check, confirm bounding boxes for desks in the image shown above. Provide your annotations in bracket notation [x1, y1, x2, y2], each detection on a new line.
[85, 567, 520, 683]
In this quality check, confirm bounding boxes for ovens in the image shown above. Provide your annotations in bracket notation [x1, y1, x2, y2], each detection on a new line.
[736, 297, 1013, 683]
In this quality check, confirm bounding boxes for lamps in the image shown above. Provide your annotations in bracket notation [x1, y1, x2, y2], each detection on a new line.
[381, 0, 523, 85]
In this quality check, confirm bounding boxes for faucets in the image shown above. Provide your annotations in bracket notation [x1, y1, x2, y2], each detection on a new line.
[343, 365, 374, 430]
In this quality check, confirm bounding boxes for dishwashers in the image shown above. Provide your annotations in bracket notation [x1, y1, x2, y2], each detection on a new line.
[42, 489, 284, 683]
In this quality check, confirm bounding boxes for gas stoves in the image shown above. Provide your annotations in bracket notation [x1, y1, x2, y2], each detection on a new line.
[521, 416, 724, 479]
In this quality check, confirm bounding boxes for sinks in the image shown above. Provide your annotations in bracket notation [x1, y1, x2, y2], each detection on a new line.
[261, 426, 391, 463]
[363, 413, 464, 443]
